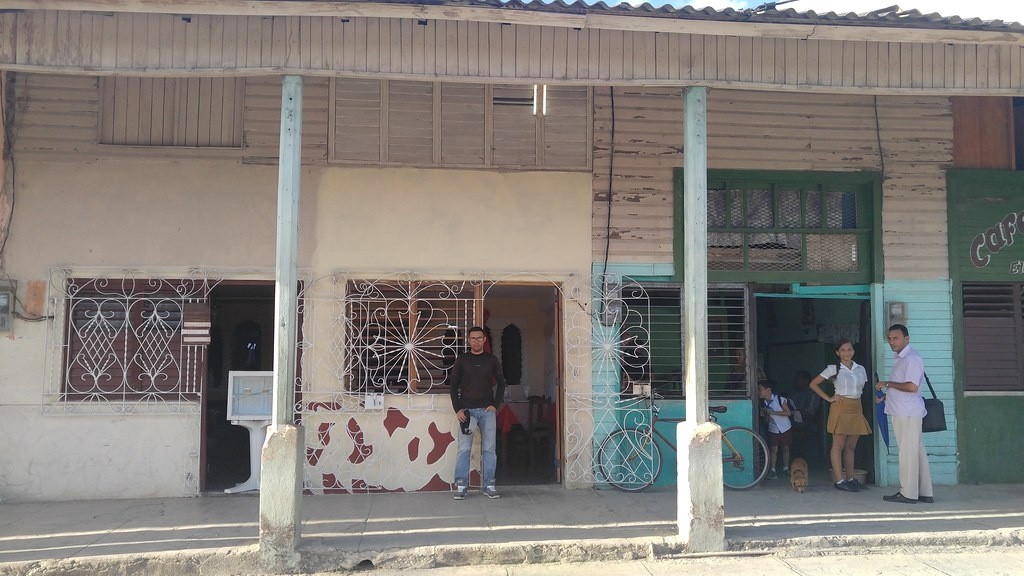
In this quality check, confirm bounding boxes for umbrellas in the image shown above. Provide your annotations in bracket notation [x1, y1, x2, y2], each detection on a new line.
[875, 371, 889, 455]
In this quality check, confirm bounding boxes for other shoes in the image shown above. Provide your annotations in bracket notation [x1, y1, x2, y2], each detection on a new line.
[780, 469, 791, 479]
[917, 496, 934, 503]
[765, 470, 778, 480]
[883, 492, 919, 504]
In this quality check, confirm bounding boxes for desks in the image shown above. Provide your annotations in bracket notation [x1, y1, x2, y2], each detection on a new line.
[496, 402, 557, 468]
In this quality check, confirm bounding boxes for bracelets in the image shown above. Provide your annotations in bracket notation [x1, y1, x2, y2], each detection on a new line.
[885, 381, 888, 386]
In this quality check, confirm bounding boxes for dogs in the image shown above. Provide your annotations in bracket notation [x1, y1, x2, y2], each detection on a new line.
[791, 458, 808, 494]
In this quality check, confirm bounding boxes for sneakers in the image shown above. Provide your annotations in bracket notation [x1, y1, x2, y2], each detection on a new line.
[453, 479, 470, 500]
[480, 480, 501, 499]
[845, 477, 869, 490]
[833, 479, 859, 492]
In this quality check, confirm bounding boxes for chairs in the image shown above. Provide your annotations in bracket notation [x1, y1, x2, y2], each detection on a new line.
[504, 395, 553, 466]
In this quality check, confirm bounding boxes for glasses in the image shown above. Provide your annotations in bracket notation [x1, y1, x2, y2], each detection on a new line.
[468, 336, 485, 342]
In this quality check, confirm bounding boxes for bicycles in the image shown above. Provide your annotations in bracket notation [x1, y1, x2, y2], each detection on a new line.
[598, 387, 770, 492]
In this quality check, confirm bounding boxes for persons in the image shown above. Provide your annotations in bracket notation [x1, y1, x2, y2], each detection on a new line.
[791, 370, 819, 416]
[625, 339, 655, 381]
[758, 381, 791, 480]
[874, 324, 934, 504]
[809, 339, 873, 491]
[450, 326, 504, 500]
[725, 346, 768, 401]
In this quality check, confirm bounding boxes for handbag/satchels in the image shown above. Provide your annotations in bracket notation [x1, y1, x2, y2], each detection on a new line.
[777, 394, 803, 430]
[921, 372, 946, 432]
